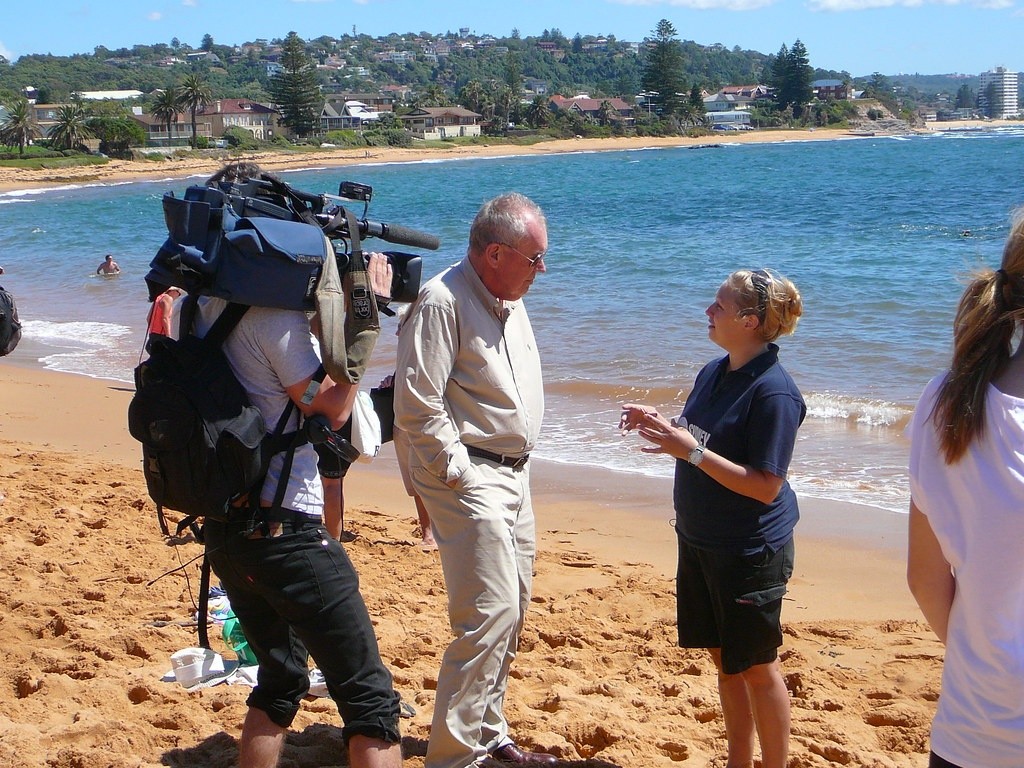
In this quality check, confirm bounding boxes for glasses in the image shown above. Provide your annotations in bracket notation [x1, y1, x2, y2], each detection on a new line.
[752, 270, 773, 324]
[485, 239, 549, 268]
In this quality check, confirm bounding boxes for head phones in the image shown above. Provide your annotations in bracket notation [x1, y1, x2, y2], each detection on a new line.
[304, 414, 361, 464]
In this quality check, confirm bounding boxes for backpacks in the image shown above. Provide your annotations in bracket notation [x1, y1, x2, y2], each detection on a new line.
[129, 294, 299, 520]
[0, 285, 21, 357]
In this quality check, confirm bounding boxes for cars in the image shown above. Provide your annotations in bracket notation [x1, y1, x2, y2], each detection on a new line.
[711, 124, 754, 131]
[204, 136, 216, 148]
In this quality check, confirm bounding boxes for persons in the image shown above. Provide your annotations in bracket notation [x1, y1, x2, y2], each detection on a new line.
[146, 287, 181, 338]
[96, 255, 120, 274]
[906, 219, 1023, 768]
[306, 302, 438, 553]
[392, 195, 555, 768]
[620, 267, 806, 768]
[169, 162, 403, 768]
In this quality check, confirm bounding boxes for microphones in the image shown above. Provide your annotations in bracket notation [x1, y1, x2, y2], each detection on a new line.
[357, 221, 440, 250]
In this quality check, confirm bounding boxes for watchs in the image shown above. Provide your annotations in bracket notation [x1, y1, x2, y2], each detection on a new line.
[687, 444, 706, 467]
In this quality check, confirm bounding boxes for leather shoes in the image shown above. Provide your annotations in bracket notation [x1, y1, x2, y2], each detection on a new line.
[478, 743, 558, 768]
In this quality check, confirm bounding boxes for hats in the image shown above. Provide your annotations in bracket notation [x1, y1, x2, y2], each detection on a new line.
[159, 646, 240, 692]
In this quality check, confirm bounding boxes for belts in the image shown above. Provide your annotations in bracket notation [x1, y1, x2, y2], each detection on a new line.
[463, 443, 530, 469]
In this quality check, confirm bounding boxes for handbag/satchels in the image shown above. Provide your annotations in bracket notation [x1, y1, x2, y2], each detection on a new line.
[222, 608, 260, 665]
